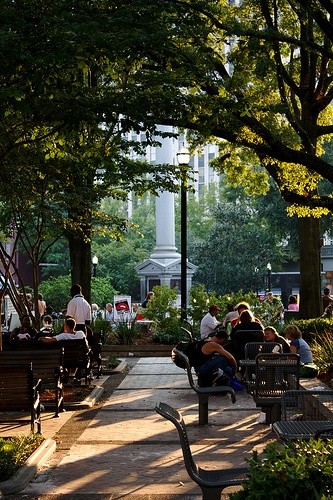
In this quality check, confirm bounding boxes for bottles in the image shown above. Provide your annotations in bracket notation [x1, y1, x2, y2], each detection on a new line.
[227, 320, 232, 330]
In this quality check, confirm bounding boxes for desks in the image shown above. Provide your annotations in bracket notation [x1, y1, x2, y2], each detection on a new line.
[239, 360, 304, 366]
[272, 421, 333, 442]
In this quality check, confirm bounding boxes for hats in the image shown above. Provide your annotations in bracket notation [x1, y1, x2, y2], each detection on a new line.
[209, 305, 221, 311]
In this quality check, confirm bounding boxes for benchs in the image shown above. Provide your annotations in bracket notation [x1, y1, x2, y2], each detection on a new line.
[0, 330, 102, 434]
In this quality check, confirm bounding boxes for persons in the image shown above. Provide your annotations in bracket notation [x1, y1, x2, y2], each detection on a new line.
[321, 287, 333, 318]
[284, 295, 299, 311]
[19, 292, 55, 341]
[64, 284, 92, 337]
[139, 292, 154, 308]
[91, 302, 114, 321]
[38, 319, 103, 364]
[263, 292, 284, 328]
[199, 302, 313, 392]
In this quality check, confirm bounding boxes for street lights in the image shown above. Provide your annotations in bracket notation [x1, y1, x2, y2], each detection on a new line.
[267, 262, 272, 292]
[176, 139, 192, 323]
[92, 255, 98, 277]
[254, 266, 259, 297]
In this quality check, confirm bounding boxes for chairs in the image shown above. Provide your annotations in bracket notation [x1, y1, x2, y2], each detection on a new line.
[155, 402, 250, 500]
[174, 349, 236, 425]
[226, 320, 333, 442]
[181, 326, 193, 341]
